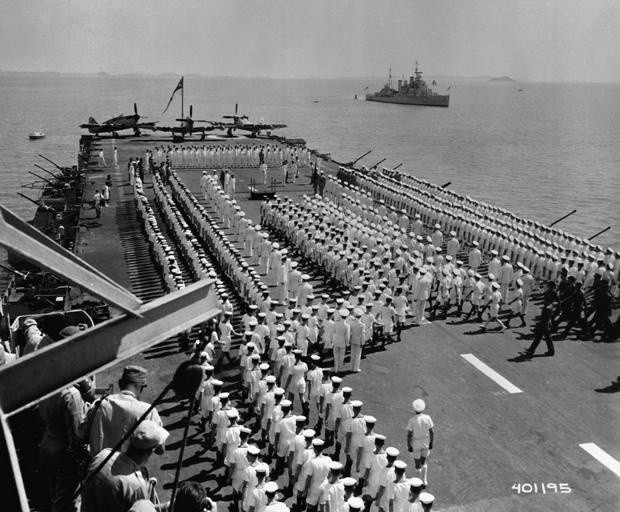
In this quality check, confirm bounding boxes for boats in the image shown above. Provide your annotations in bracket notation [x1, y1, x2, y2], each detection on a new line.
[28, 130, 46, 139]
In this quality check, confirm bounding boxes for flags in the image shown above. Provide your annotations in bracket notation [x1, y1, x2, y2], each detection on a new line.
[163, 76, 183, 113]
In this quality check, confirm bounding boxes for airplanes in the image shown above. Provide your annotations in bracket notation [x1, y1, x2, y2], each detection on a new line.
[156, 104, 225, 141]
[78, 101, 160, 139]
[210, 100, 288, 138]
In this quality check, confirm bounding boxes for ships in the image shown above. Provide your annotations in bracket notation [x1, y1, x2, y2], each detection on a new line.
[365, 57, 450, 107]
[0, 76, 620, 512]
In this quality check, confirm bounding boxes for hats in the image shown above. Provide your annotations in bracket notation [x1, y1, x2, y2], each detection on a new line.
[133, 169, 314, 335]
[503, 211, 529, 285]
[133, 420, 170, 449]
[581, 238, 619, 270]
[146, 144, 307, 153]
[462, 196, 500, 289]
[316, 172, 459, 317]
[123, 366, 147, 383]
[201, 337, 435, 508]
[528, 220, 580, 262]
